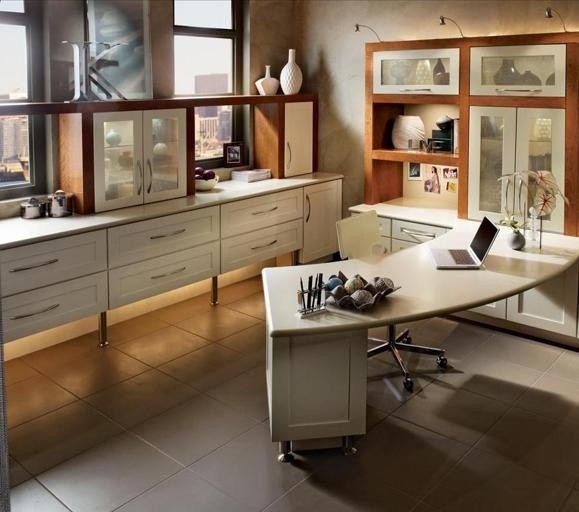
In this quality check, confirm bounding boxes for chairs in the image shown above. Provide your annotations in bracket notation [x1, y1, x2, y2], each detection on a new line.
[335, 209, 447, 403]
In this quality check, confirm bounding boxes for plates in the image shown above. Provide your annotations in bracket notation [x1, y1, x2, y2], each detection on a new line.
[232, 168, 270, 183]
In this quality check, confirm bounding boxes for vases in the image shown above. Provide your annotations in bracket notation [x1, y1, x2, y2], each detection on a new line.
[280, 49, 302, 95]
[255, 65, 279, 96]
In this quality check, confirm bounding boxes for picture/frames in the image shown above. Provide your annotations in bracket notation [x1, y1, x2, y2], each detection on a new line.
[85, 0, 153, 99]
[223, 142, 246, 167]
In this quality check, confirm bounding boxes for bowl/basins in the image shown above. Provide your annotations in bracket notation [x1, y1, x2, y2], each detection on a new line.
[195, 175, 219, 191]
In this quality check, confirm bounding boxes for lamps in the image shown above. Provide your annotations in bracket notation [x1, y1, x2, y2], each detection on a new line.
[355, 24, 383, 43]
[440, 15, 464, 38]
[545, 7, 567, 32]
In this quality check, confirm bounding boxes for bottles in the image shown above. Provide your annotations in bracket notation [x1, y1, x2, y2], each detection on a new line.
[105, 127, 123, 146]
[117, 149, 134, 169]
[433, 58, 445, 74]
[491, 57, 521, 85]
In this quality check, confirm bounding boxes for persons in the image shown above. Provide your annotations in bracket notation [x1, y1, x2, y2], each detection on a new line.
[424, 166, 440, 194]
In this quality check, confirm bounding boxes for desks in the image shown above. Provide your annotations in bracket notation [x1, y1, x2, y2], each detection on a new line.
[262, 227, 578, 462]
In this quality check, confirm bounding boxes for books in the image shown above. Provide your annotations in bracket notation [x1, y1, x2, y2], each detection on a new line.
[231, 168, 272, 183]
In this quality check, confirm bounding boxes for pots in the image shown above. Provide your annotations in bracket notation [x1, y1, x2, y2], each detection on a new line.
[20, 198, 47, 219]
[48, 188, 75, 218]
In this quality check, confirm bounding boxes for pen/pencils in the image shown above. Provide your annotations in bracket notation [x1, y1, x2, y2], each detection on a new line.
[300, 272, 323, 315]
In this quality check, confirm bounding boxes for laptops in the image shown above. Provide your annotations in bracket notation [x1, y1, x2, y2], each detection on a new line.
[430, 215, 500, 269]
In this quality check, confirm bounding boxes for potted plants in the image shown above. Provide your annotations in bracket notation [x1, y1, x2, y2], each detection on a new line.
[499, 215, 529, 250]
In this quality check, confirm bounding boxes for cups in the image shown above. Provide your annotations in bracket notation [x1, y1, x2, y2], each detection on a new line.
[433, 72, 449, 85]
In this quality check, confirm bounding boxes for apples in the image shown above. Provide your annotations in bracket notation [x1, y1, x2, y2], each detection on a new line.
[195, 167, 215, 179]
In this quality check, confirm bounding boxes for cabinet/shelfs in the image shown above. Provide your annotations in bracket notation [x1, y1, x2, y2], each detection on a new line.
[464, 32, 577, 237]
[254, 93, 315, 176]
[106, 203, 221, 314]
[0, 214, 107, 345]
[220, 186, 304, 273]
[466, 257, 579, 339]
[351, 212, 451, 254]
[298, 177, 344, 268]
[55, 99, 193, 213]
[365, 39, 464, 220]
